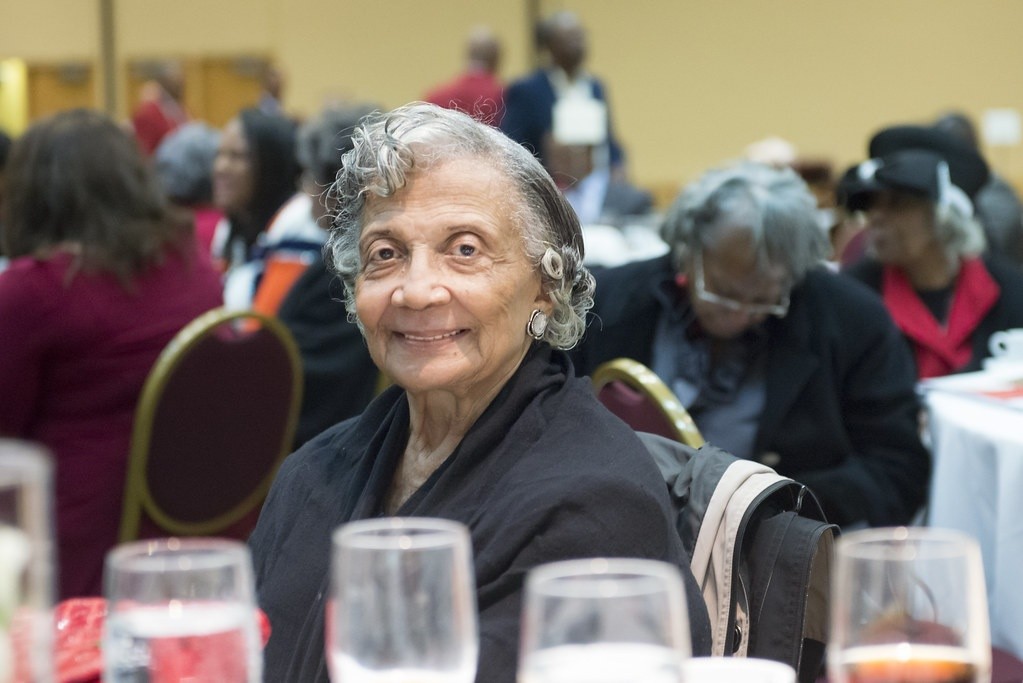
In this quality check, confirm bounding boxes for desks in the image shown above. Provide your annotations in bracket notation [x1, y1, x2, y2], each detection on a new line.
[904, 369, 1023, 664]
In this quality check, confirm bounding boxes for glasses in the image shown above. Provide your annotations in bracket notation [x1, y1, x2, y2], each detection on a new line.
[693, 250, 792, 320]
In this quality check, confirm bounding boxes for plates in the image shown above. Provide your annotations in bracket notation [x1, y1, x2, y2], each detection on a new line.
[692, 656, 796, 683]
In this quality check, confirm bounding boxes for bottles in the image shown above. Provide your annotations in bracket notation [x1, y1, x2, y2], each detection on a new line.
[516, 558, 692, 683]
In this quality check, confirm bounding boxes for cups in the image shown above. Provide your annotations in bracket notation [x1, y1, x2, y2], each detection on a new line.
[0, 439, 61, 683]
[825, 526, 993, 682]
[100, 537, 263, 683]
[988, 328, 1022, 358]
[325, 516, 479, 683]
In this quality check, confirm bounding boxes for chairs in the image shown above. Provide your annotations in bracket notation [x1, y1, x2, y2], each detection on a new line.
[591, 355, 708, 450]
[117, 304, 303, 543]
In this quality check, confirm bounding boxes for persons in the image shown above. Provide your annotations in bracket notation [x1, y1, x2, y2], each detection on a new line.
[424, 11, 625, 193]
[244, 101, 713, 683]
[0, 56, 391, 603]
[792, 112, 1023, 381]
[571, 161, 933, 540]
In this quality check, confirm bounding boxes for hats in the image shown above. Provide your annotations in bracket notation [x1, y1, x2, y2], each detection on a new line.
[837, 149, 950, 210]
[870, 115, 990, 194]
